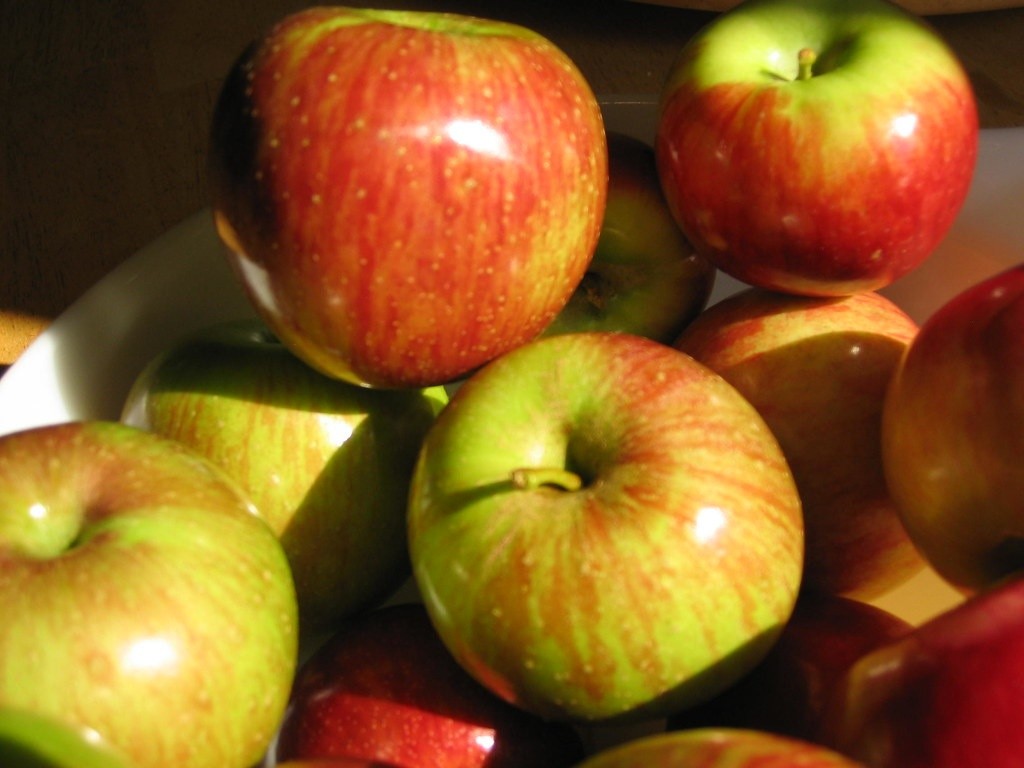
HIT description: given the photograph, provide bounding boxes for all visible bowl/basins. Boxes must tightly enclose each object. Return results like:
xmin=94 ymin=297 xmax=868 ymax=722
xmin=0 ymin=133 xmax=1024 ymax=768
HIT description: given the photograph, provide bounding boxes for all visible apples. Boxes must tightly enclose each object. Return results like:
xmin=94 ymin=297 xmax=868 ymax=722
xmin=0 ymin=0 xmax=1024 ymax=768
